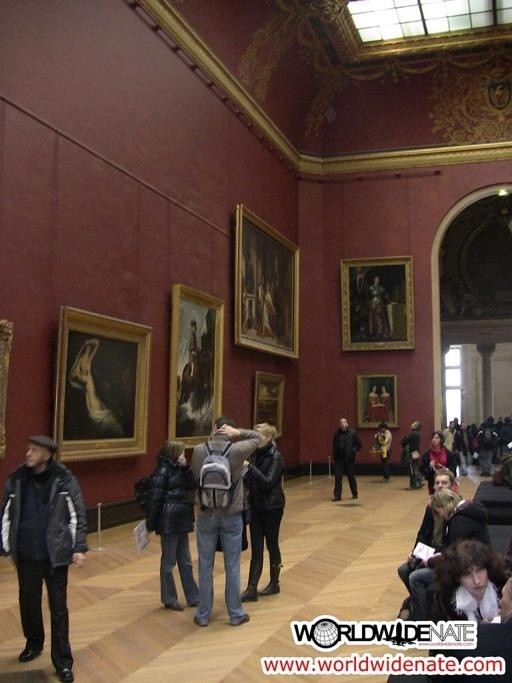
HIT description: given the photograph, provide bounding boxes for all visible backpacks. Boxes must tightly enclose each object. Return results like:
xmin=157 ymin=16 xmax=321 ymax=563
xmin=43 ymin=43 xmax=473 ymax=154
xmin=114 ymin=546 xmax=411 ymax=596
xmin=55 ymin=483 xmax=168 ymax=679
xmin=199 ymin=440 xmax=234 ymax=508
xmin=483 ymin=427 xmax=493 ymax=446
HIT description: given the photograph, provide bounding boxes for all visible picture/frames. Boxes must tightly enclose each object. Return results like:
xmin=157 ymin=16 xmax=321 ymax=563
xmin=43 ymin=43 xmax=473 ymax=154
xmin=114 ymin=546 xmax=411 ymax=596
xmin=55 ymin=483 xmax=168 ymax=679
xmin=167 ymin=283 xmax=226 ymax=446
xmin=252 ymin=369 xmax=286 ymax=439
xmin=339 ymin=255 xmax=416 ymax=354
xmin=233 ymin=203 xmax=301 ymax=360
xmin=0 ymin=320 xmax=15 ymax=459
xmin=52 ymin=305 xmax=153 ymax=463
xmin=356 ymin=373 xmax=399 ymax=430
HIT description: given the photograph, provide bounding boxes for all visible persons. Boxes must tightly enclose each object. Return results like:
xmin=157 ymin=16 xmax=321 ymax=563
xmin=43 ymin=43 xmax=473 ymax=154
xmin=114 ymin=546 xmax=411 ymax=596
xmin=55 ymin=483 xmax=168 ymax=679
xmin=0 ymin=435 xmax=89 ymax=682
xmin=145 ymin=440 xmax=204 ymax=611
xmin=330 ymin=417 xmax=362 ymax=501
xmin=235 ymin=423 xmax=285 ymax=601
xmin=387 ymin=415 xmax=512 ymax=683
xmin=358 ymin=274 xmax=393 ymax=338
xmin=67 ymin=338 xmax=126 ymax=439
xmin=381 ymin=385 xmax=393 ymax=421
xmin=188 ymin=320 xmax=200 ymax=377
xmin=374 ymin=423 xmax=392 ymax=482
xmin=367 ymin=385 xmax=380 ymax=420
xmin=191 ymin=417 xmax=263 ymax=626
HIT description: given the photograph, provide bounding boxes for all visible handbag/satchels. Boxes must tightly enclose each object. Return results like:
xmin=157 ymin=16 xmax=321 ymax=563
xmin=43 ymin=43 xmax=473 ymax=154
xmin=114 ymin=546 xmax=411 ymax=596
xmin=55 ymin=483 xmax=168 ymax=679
xmin=410 ymin=450 xmax=420 ymax=460
xmin=134 ymin=473 xmax=151 ymax=512
xmin=493 ymin=469 xmax=504 ymax=485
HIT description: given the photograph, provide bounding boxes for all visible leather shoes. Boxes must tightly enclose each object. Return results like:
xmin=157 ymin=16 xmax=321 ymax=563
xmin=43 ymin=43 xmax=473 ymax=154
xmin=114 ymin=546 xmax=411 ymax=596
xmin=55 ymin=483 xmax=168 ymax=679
xmin=56 ymin=668 xmax=74 ymax=682
xmin=20 ymin=646 xmax=43 ymax=661
xmin=164 ymin=601 xmax=200 ymax=610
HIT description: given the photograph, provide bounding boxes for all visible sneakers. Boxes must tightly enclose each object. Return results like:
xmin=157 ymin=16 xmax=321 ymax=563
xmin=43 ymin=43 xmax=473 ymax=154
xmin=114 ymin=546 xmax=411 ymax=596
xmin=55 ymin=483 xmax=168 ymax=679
xmin=407 ymin=480 xmax=425 ymax=490
xmin=331 ymin=497 xmax=341 ymax=501
xmin=479 ymin=473 xmax=492 ymax=476
xmin=194 ymin=616 xmax=207 ymax=626
xmin=231 ymin=613 xmax=249 ymax=626
xmin=353 ymin=494 xmax=357 ymax=499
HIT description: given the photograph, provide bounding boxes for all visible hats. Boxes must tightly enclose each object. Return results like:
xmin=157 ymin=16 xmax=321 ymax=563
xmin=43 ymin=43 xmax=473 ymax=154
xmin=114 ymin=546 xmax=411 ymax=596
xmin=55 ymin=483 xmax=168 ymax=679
xmin=26 ymin=435 xmax=58 ymax=452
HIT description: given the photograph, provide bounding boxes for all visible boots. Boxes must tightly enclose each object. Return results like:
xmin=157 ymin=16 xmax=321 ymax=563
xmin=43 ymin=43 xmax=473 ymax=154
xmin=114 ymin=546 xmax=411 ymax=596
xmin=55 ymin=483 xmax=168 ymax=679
xmin=258 ymin=557 xmax=283 ymax=595
xmin=240 ymin=558 xmax=263 ymax=602
